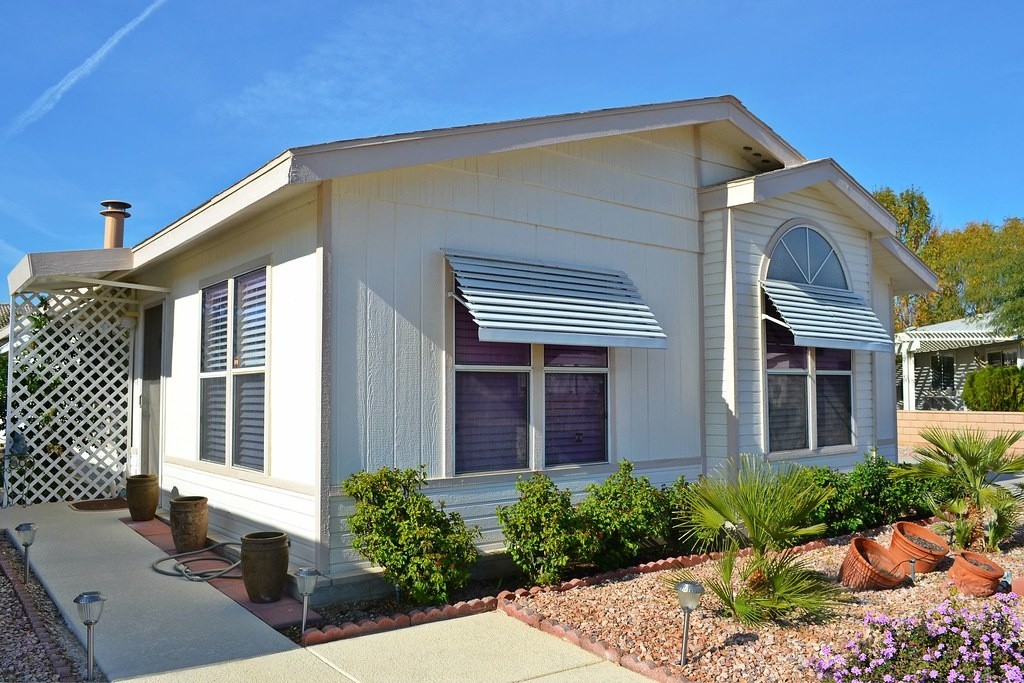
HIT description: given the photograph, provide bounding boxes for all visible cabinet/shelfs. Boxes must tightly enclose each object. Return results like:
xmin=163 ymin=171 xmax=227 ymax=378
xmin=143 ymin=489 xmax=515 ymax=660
xmin=2 ymin=454 xmax=35 ymax=508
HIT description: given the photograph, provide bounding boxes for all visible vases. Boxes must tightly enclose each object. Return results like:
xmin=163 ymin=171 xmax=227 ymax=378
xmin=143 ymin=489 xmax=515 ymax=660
xmin=838 ymin=537 xmax=905 ymax=590
xmin=890 ymin=522 xmax=950 ymax=573
xmin=947 ymin=552 xmax=1005 ymax=597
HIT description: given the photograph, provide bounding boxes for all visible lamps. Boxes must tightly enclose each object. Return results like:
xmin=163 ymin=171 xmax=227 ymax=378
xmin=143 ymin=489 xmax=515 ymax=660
xmin=672 ymin=580 xmax=704 ymax=666
xmin=15 ymin=523 xmax=41 ymax=583
xmin=293 ymin=565 xmax=320 ymax=635
xmin=73 ymin=591 xmax=108 ymax=682
